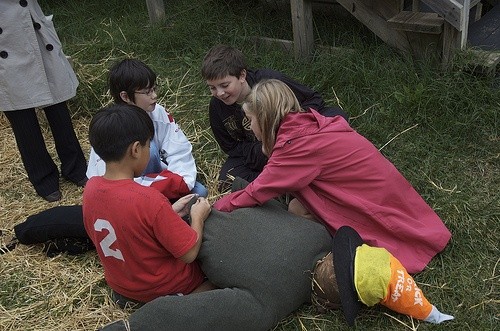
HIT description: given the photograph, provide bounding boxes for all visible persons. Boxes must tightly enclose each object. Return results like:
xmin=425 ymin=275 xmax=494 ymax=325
xmin=85 ymin=57 xmax=208 ymax=206
xmin=211 ymin=79 xmax=451 ymax=273
xmin=201 ymin=44 xmax=352 ymax=204
xmin=80 ymin=102 xmax=212 ymax=310
xmin=0 ymin=0 xmax=89 ymax=203
xmin=14 ymin=185 xmax=391 ymax=331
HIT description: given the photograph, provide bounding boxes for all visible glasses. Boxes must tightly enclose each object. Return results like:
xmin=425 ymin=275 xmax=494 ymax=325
xmin=133 ymin=84 xmax=160 ymax=96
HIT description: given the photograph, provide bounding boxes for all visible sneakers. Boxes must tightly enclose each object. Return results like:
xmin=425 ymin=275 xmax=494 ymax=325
xmin=76 ymin=177 xmax=88 ymax=188
xmin=46 ymin=189 xmax=62 ymax=201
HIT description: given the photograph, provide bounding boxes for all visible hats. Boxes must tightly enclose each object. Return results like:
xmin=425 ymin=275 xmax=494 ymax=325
xmin=332 ymin=225 xmax=455 ymax=327
